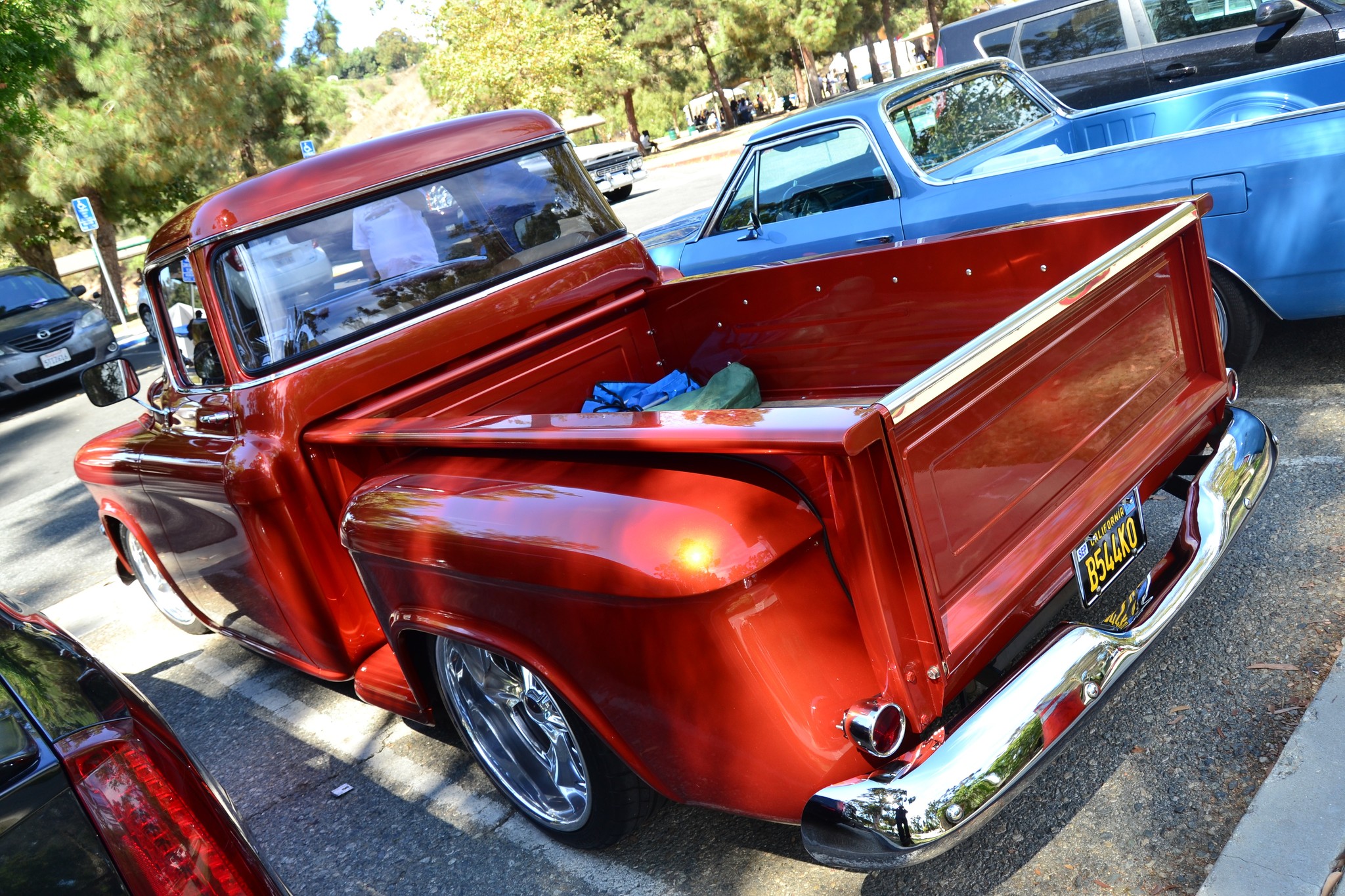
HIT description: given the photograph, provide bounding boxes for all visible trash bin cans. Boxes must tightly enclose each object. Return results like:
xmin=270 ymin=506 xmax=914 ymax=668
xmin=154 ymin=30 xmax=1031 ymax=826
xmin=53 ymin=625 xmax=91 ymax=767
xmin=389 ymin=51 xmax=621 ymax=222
xmin=688 ymin=125 xmax=696 ymax=135
xmin=668 ymin=128 xmax=678 ymax=140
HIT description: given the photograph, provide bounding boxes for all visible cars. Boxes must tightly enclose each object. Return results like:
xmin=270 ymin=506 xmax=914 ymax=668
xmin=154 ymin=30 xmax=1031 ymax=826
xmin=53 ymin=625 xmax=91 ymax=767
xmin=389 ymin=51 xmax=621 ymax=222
xmin=0 ymin=596 xmax=293 ymax=895
xmin=430 ymin=141 xmax=649 ymax=229
xmin=136 ymin=234 xmax=337 ymax=347
xmin=0 ymin=266 xmax=122 ymax=396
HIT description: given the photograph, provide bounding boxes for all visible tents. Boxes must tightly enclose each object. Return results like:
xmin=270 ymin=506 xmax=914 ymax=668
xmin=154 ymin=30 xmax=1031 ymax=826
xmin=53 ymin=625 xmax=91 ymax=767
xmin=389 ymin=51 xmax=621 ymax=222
xmin=830 ymin=19 xmax=948 ymax=84
xmin=559 ymin=109 xmax=606 ymax=143
xmin=46 ymin=232 xmax=152 ymax=278
xmin=682 ymin=71 xmax=773 ymax=113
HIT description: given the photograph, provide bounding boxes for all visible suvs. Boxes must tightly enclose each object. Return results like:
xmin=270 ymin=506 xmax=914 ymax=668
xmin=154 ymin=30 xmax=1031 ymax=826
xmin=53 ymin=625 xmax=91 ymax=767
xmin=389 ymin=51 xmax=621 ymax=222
xmin=933 ymin=0 xmax=1344 ymax=144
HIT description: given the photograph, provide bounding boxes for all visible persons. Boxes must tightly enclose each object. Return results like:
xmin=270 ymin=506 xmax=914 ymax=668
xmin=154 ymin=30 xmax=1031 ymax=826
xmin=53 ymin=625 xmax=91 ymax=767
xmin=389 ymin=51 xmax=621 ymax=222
xmin=910 ymin=48 xmax=942 ymax=70
xmin=814 ymin=64 xmax=854 ymax=100
xmin=61 ymin=263 xmax=144 ymax=311
xmin=352 ymin=194 xmax=440 ymax=284
xmin=688 ymin=92 xmax=767 ymax=133
xmin=638 ymin=128 xmax=663 ymax=155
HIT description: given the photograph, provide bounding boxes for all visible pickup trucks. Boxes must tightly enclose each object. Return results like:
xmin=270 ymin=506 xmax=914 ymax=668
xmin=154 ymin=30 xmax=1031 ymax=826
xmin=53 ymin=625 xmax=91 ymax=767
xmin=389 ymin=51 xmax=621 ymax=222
xmin=75 ymin=118 xmax=1281 ymax=874
xmin=644 ymin=57 xmax=1343 ymax=379
